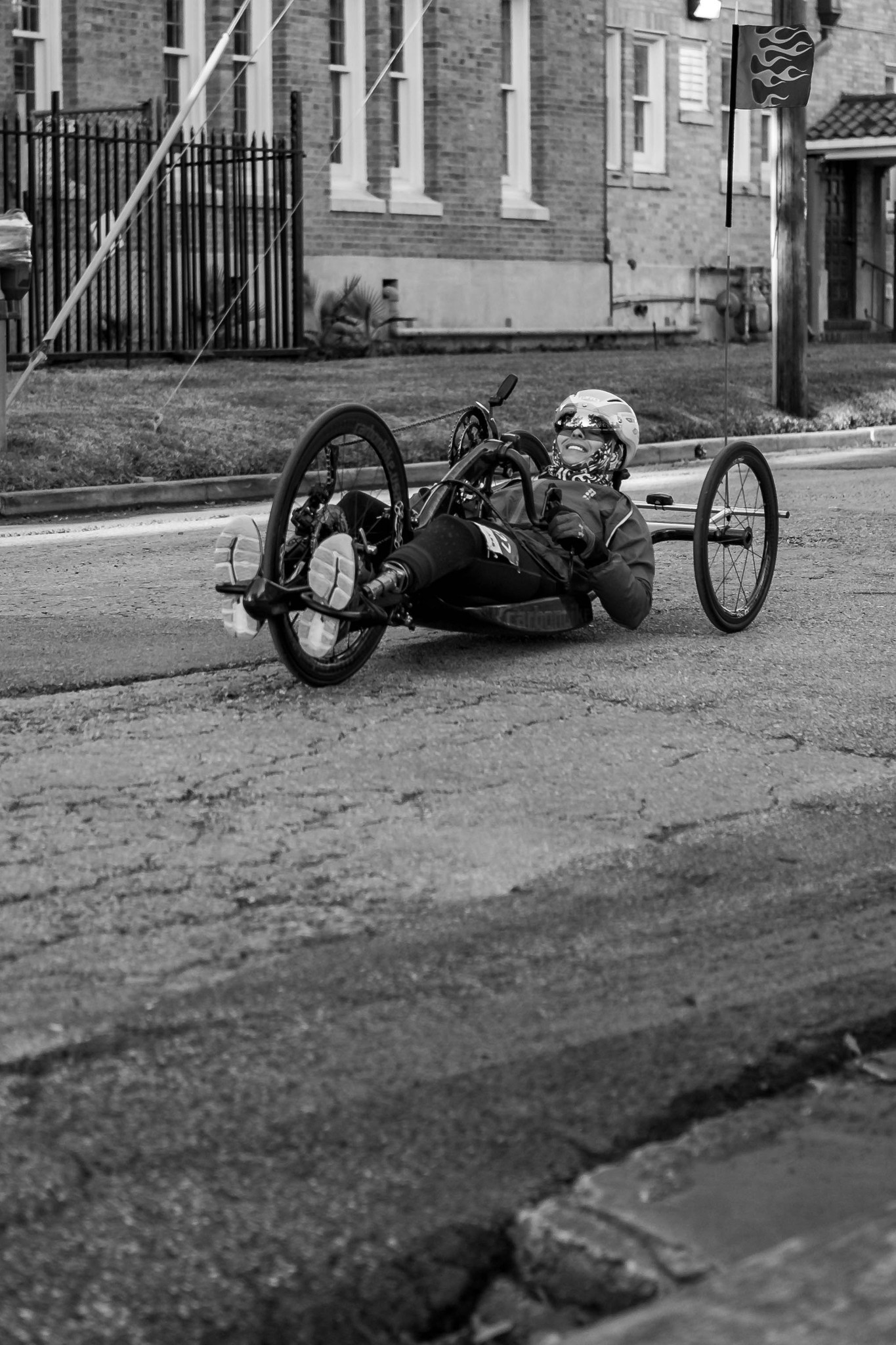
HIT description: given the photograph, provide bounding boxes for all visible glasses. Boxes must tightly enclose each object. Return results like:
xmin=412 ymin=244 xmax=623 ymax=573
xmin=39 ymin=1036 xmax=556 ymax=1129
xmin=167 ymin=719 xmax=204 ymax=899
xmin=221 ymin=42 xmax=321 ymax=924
xmin=561 ymin=419 xmax=606 ymax=437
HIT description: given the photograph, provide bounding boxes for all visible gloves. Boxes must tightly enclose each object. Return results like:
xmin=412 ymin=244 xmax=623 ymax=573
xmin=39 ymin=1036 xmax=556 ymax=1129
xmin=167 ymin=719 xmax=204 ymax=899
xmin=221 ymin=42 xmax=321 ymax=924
xmin=543 ymin=506 xmax=608 ymax=567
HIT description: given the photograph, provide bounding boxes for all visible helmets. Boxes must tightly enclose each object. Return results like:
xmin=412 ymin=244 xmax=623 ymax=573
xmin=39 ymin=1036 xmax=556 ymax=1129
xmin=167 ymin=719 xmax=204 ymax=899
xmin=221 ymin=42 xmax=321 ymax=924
xmin=553 ymin=389 xmax=640 ymax=472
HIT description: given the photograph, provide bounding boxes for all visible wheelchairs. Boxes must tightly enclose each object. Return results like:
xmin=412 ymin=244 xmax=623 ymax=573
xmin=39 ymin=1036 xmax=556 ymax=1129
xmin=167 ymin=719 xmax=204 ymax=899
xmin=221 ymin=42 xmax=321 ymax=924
xmin=215 ymin=373 xmax=790 ymax=689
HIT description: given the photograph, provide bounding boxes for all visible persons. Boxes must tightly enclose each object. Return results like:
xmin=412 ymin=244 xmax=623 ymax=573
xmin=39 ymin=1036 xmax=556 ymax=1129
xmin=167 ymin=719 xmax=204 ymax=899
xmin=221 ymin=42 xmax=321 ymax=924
xmin=215 ymin=389 xmax=656 ymax=659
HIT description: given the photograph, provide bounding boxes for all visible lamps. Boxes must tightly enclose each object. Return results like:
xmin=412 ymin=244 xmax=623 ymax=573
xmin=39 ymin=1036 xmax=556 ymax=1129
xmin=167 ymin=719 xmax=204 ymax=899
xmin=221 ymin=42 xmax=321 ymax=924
xmin=686 ymin=0 xmax=722 ymax=21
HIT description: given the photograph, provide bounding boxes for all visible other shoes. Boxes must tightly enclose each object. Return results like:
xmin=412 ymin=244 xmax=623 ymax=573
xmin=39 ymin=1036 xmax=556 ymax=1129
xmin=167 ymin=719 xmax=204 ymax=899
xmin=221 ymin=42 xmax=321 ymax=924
xmin=213 ymin=516 xmax=268 ymax=640
xmin=297 ymin=532 xmax=358 ymax=657
